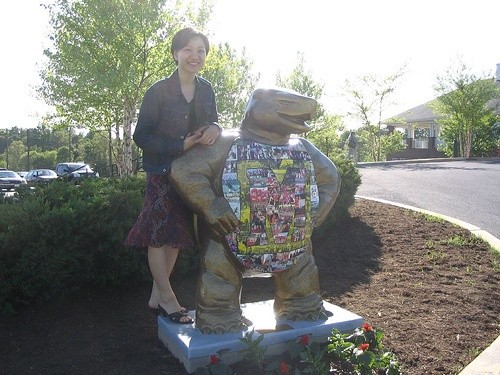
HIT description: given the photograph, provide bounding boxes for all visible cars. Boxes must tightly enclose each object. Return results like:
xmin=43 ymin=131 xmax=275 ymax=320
xmin=25 ymin=168 xmax=63 ymax=186
xmin=0 ymin=171 xmax=27 ymax=192
xmin=16 ymin=172 xmax=30 ymax=178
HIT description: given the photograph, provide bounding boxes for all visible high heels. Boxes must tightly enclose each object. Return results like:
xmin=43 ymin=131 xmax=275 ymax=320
xmin=147 ymin=304 xmax=194 ymax=324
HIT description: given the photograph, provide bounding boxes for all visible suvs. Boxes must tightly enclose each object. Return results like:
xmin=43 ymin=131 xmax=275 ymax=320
xmin=53 ymin=163 xmax=101 ymax=184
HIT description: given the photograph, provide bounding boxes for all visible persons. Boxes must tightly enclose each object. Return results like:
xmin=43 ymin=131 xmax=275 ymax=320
xmin=130 ymin=26 xmax=223 ymax=327
